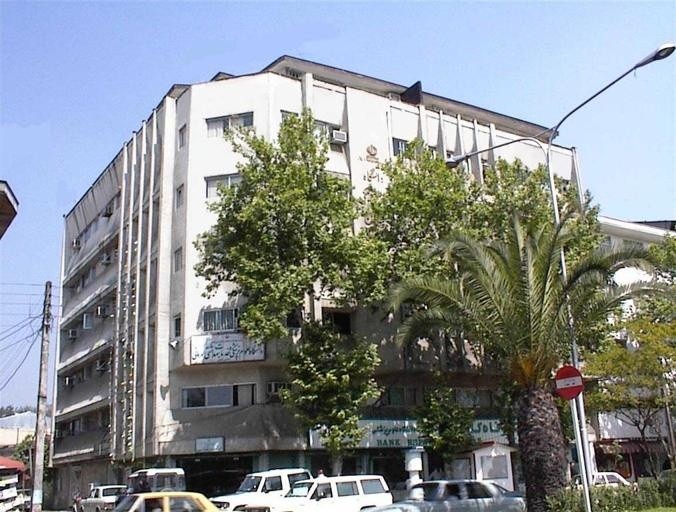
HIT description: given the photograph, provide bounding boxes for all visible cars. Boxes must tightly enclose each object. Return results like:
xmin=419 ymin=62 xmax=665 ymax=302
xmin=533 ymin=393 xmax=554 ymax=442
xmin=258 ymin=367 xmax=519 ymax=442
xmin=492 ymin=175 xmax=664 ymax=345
xmin=563 ymin=471 xmax=639 ymax=498
xmin=361 ymin=479 xmax=526 ymax=512
xmin=77 ymin=485 xmax=127 ymax=512
xmin=108 ymin=491 xmax=222 ymax=512
xmin=658 ymin=468 xmax=676 ymax=480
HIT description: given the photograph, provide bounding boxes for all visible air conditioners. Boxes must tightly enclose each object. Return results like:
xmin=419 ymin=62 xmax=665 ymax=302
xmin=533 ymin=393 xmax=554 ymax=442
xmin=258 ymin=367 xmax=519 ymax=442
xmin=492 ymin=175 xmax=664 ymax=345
xmin=329 ymin=129 xmax=348 ymax=145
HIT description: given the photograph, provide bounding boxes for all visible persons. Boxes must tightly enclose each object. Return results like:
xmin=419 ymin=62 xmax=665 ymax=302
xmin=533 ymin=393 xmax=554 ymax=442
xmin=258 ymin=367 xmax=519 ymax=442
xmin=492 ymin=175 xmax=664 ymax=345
xmin=114 ymin=479 xmax=151 ymax=506
xmin=428 ymin=466 xmax=442 ymax=480
xmin=316 ymin=468 xmax=325 ymax=477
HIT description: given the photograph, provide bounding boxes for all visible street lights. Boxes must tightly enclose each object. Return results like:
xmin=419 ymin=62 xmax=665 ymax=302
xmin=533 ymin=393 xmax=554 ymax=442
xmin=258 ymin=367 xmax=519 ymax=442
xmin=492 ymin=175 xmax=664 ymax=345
xmin=445 ymin=42 xmax=675 ymax=507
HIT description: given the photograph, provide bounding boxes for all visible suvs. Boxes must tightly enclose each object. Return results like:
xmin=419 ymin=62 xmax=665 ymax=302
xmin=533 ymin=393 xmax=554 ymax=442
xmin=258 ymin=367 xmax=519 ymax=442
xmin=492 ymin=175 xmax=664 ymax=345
xmin=207 ymin=467 xmax=314 ymax=512
xmin=115 ymin=468 xmax=186 ymax=509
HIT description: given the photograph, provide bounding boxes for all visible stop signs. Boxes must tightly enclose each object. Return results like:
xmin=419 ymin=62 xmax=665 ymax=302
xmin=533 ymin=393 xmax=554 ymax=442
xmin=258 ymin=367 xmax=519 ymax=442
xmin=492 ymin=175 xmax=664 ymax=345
xmin=555 ymin=366 xmax=584 ymax=401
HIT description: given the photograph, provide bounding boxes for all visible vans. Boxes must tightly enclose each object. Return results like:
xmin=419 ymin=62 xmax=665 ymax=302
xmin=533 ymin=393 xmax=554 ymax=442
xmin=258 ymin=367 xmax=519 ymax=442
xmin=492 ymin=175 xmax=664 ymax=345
xmin=244 ymin=474 xmax=393 ymax=511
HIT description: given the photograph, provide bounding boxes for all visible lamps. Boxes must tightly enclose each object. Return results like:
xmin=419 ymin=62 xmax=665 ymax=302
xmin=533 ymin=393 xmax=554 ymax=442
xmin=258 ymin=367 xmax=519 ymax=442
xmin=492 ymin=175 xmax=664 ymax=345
xmin=167 ymin=338 xmax=181 ymax=351
